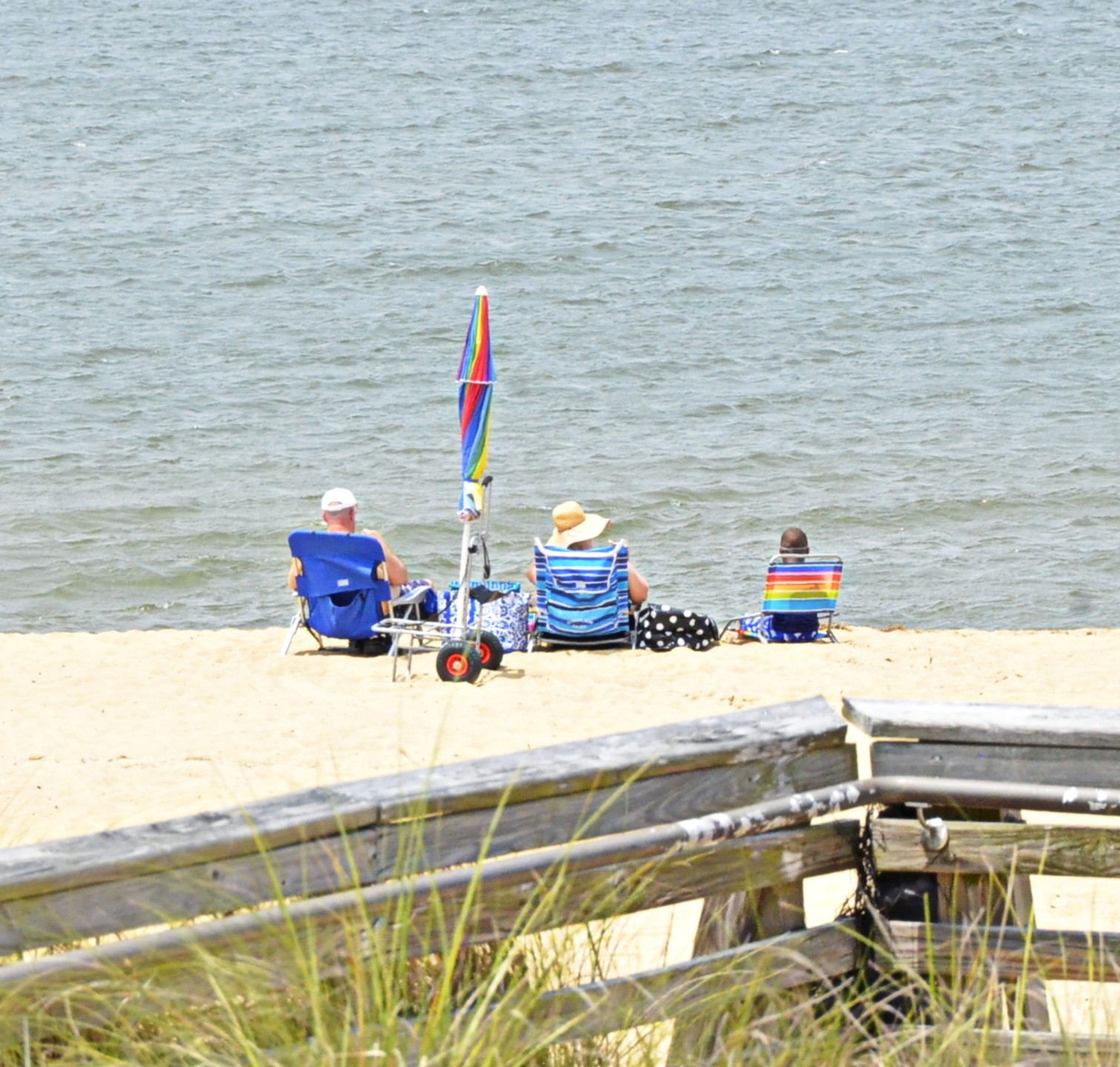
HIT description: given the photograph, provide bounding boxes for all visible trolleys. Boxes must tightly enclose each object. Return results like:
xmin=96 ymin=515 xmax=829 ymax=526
xmin=373 ymin=477 xmax=504 ymax=684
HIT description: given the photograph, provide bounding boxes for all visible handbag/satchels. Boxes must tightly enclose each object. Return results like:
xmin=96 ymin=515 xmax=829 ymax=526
xmin=637 ymin=603 xmax=720 ymax=652
xmin=437 ymin=581 xmax=532 ymax=654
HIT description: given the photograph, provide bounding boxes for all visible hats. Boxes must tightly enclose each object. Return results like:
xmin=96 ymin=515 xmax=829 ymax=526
xmin=543 ymin=500 xmax=612 ymax=549
xmin=321 ymin=488 xmax=356 ymax=512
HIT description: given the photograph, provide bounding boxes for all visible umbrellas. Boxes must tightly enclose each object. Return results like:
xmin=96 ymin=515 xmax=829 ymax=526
xmin=450 ymin=285 xmax=497 ymax=644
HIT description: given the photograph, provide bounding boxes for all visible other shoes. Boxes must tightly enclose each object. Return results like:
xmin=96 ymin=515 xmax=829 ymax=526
xmin=349 ymin=637 xmax=403 ymax=657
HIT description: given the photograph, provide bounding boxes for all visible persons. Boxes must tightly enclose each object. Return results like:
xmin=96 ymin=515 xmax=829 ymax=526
xmin=735 ymin=527 xmax=819 ymax=641
xmin=287 ymin=488 xmax=436 ymax=626
xmin=526 ymin=500 xmax=649 ymax=641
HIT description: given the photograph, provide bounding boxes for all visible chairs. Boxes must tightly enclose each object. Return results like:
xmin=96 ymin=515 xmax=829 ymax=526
xmin=278 ymin=530 xmax=432 ymax=652
xmin=719 ymin=553 xmax=844 ymax=642
xmin=532 ymin=535 xmax=638 ymax=651
